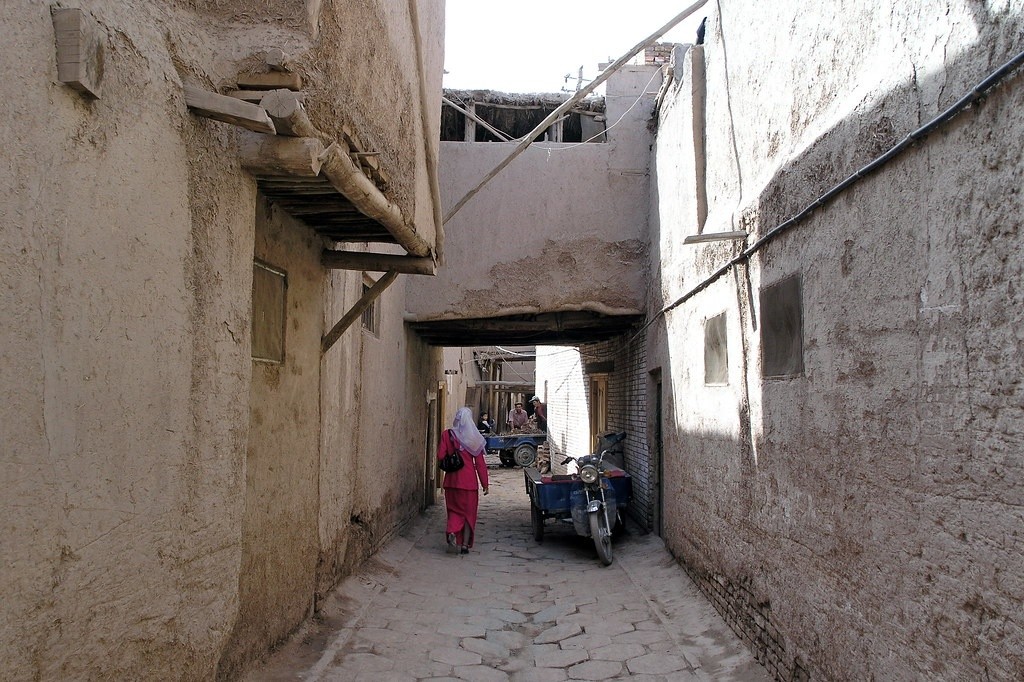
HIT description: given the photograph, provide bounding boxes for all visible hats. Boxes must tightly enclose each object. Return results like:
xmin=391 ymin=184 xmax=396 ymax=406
xmin=514 ymin=402 xmax=523 ymax=405
xmin=529 ymin=396 xmax=539 ymax=402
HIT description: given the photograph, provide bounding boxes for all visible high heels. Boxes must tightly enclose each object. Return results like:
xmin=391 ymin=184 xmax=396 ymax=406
xmin=461 ymin=545 xmax=469 ymax=554
xmin=447 ymin=534 xmax=455 ymax=547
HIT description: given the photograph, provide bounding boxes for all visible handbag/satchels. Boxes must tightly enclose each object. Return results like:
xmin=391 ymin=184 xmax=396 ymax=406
xmin=438 ymin=429 xmax=464 ymax=471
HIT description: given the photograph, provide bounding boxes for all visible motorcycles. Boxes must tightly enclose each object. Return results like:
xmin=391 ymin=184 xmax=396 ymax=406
xmin=523 ymin=450 xmax=632 ymax=566
xmin=480 ymin=430 xmax=548 ymax=467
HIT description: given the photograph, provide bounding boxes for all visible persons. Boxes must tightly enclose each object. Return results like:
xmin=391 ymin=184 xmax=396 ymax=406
xmin=438 ymin=407 xmax=488 ymax=555
xmin=509 ymin=402 xmax=528 ymax=434
xmin=477 ymin=411 xmax=491 ymax=433
xmin=529 ymin=396 xmax=547 ymax=433
